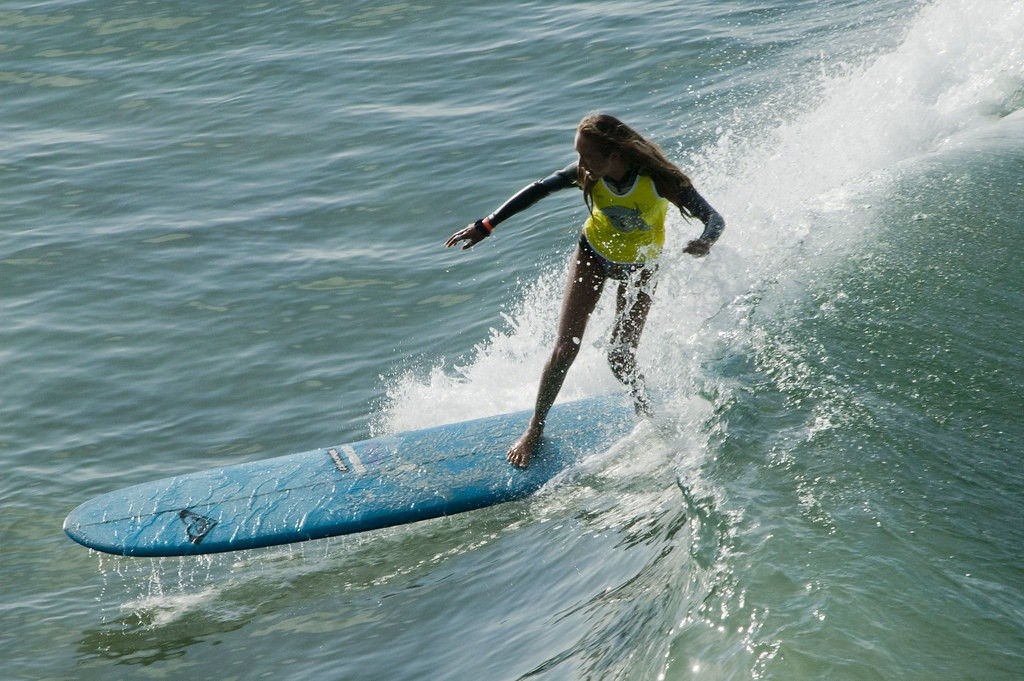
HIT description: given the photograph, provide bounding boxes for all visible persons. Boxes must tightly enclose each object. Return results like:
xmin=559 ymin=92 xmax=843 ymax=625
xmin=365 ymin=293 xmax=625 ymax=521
xmin=444 ymin=114 xmax=726 ymax=471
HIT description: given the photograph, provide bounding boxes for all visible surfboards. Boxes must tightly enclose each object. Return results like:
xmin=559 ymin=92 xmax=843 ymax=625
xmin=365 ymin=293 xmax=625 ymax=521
xmin=64 ymin=388 xmax=659 ymax=552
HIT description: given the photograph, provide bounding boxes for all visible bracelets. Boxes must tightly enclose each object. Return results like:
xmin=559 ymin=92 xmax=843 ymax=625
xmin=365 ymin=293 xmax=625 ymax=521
xmin=475 ymin=219 xmax=491 ymax=237
xmin=482 ymin=219 xmax=493 ymax=231
